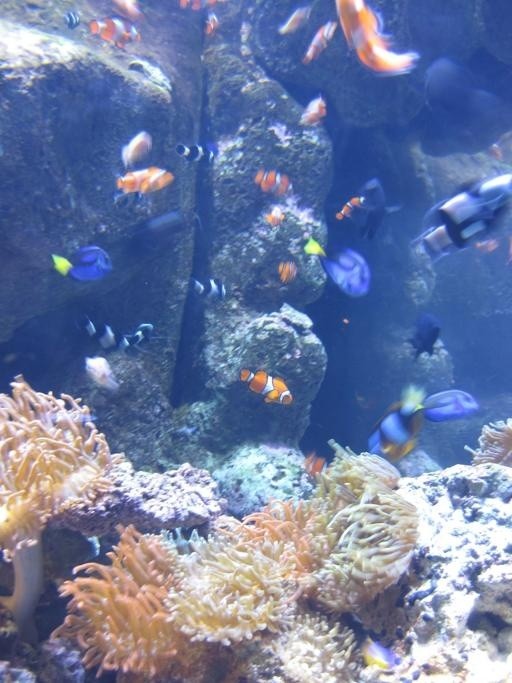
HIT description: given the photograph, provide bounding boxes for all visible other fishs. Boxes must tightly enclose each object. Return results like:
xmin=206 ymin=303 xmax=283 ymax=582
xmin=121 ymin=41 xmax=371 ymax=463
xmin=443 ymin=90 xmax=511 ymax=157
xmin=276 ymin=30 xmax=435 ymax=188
xmin=50 ymin=246 xmax=111 ymax=283
xmin=194 ymin=280 xmax=232 ymax=301
xmin=84 ymin=358 xmax=118 ymax=393
xmin=237 ymin=371 xmax=292 ymax=408
xmin=277 ymin=263 xmax=300 ymax=287
xmin=304 ymin=240 xmax=369 ymax=299
xmin=80 ymin=317 xmax=153 ymax=353
xmin=48 ymin=0 xmax=420 ymax=249
